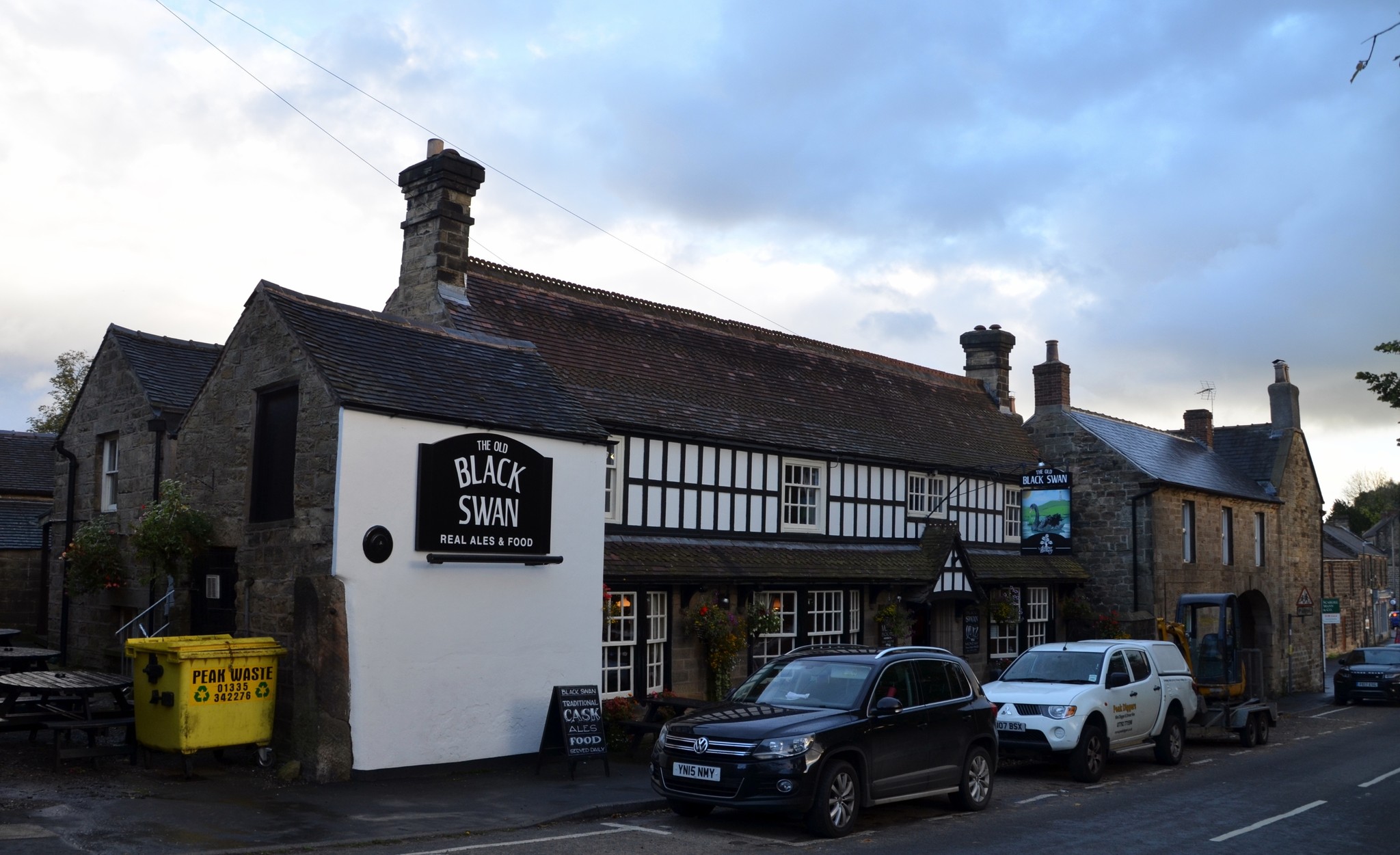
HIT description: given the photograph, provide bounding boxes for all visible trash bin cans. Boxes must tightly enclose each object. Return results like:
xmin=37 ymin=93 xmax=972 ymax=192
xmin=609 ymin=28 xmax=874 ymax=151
xmin=124 ymin=631 xmax=288 ymax=753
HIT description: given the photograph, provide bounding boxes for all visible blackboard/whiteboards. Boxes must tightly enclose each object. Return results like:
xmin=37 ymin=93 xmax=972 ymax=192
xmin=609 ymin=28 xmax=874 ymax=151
xmin=539 ymin=685 xmax=609 ymax=763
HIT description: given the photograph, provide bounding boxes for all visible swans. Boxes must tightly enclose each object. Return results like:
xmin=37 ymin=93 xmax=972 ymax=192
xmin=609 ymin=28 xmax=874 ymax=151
xmin=1028 ymin=501 xmax=1066 ymax=533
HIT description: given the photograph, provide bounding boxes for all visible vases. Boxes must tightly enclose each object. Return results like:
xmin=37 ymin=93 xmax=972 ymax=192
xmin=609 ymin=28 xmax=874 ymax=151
xmin=754 ymin=618 xmax=770 ymax=629
xmin=1065 ymin=613 xmax=1080 ymax=619
xmin=1082 ymin=609 xmax=1096 ymax=621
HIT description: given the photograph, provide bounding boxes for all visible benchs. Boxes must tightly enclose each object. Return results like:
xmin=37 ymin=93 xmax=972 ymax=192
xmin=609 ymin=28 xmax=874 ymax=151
xmin=0 ymin=693 xmax=137 ymax=765
xmin=618 ymin=718 xmax=661 ymax=732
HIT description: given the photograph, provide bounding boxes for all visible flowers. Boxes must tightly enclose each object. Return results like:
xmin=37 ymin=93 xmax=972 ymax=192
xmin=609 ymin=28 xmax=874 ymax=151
xmin=54 ymin=514 xmax=128 ymax=603
xmin=605 ymin=696 xmax=640 ymax=749
xmin=123 ymin=474 xmax=215 ymax=592
xmin=682 ymin=594 xmax=747 ymax=701
xmin=1063 ymin=593 xmax=1096 ymax=612
xmin=602 ymin=583 xmax=620 ymax=626
xmin=869 ymin=599 xmax=917 ymax=646
xmin=990 ymin=585 xmax=1025 ymax=628
xmin=748 ymin=599 xmax=784 ymax=639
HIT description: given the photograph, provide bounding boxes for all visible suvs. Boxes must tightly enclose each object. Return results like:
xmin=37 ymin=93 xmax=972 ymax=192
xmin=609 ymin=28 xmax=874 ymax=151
xmin=648 ymin=643 xmax=1000 ymax=839
xmin=981 ymin=639 xmax=1200 ymax=784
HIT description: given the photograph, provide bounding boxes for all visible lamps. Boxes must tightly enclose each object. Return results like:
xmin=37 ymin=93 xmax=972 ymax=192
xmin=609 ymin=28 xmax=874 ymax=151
xmin=616 ymin=594 xmax=631 ymax=608
xmin=773 ymin=598 xmax=783 ymax=609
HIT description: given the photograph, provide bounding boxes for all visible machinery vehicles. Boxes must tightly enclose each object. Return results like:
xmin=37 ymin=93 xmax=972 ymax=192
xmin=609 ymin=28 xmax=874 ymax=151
xmin=1154 ymin=580 xmax=1279 ymax=749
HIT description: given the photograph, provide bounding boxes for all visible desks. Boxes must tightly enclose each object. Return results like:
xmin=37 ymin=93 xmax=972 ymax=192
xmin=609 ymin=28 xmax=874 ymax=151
xmin=625 ymin=693 xmax=713 ymax=758
xmin=0 ymin=669 xmax=133 ymax=763
xmin=0 ymin=644 xmax=62 ymax=673
xmin=0 ymin=628 xmax=21 ymax=646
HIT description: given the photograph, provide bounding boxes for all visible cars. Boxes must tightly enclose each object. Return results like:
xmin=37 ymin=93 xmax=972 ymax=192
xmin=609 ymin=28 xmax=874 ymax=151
xmin=1332 ymin=643 xmax=1400 ymax=708
xmin=1388 ymin=610 xmax=1400 ymax=630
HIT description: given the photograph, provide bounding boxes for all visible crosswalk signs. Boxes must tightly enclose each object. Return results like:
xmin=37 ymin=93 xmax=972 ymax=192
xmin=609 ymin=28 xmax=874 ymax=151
xmin=1295 ymin=585 xmax=1314 ymax=607
